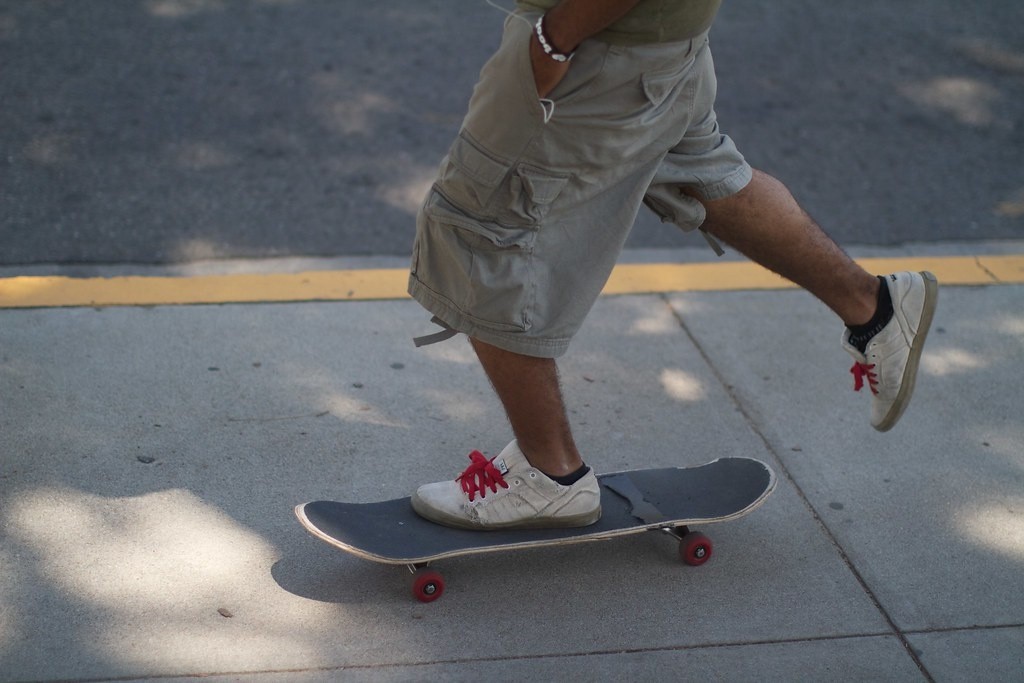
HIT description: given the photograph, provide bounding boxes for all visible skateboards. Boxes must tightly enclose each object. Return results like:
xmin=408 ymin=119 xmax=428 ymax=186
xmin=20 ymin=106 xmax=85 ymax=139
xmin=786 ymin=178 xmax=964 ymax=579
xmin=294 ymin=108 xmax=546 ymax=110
xmin=292 ymin=456 xmax=781 ymax=605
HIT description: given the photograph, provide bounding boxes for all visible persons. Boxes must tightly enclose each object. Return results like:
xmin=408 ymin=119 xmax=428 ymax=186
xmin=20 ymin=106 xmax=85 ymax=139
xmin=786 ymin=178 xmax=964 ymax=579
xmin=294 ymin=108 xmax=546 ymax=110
xmin=407 ymin=0 xmax=938 ymax=530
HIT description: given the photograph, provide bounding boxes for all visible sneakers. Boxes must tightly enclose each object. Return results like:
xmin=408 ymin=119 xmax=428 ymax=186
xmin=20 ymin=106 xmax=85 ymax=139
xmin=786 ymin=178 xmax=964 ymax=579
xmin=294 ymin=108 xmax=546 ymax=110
xmin=412 ymin=438 xmax=603 ymax=530
xmin=842 ymin=270 xmax=939 ymax=431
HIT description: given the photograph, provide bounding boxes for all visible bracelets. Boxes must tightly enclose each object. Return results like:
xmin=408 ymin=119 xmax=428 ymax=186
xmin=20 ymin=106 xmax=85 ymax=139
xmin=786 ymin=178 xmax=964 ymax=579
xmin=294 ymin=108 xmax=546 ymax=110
xmin=534 ymin=12 xmax=575 ymax=63
